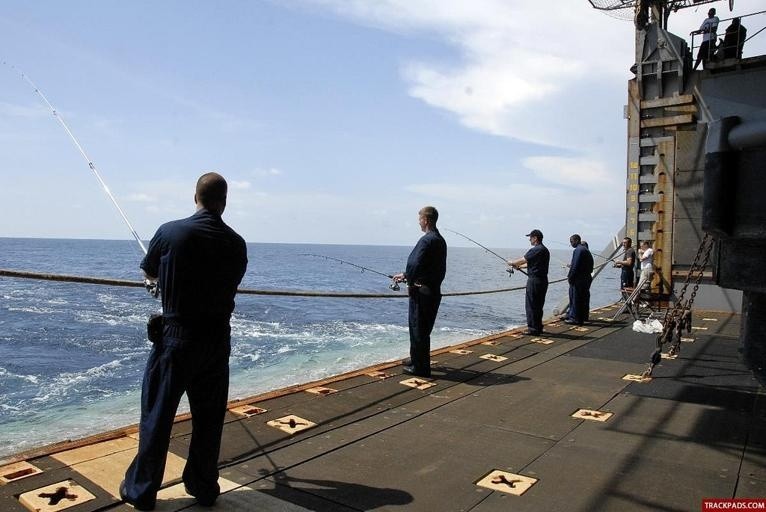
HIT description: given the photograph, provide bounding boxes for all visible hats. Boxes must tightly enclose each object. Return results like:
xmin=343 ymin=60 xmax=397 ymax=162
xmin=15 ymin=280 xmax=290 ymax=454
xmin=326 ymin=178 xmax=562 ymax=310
xmin=526 ymin=229 xmax=543 ymax=238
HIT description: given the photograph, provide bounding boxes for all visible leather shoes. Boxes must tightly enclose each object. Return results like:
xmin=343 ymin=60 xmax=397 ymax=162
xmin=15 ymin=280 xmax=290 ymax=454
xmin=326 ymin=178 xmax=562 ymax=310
xmin=402 ymin=365 xmax=431 ymax=378
xmin=119 ymin=479 xmax=156 ymax=511
xmin=185 ymin=486 xmax=217 ymax=506
xmin=559 ymin=316 xmax=569 ymax=321
xmin=522 ymin=330 xmax=540 ymax=336
xmin=564 ymin=319 xmax=580 ymax=324
xmin=402 ymin=358 xmax=412 ymax=366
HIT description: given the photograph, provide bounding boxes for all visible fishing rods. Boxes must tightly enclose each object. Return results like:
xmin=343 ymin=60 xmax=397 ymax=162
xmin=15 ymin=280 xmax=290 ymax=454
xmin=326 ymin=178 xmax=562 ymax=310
xmin=0 ymin=59 xmax=162 ymax=295
xmin=227 ymin=407 xmax=288 ymax=480
xmin=591 ymin=251 xmax=614 ymax=263
xmin=301 ymin=254 xmax=409 ymax=292
xmin=443 ymin=228 xmax=530 ymax=276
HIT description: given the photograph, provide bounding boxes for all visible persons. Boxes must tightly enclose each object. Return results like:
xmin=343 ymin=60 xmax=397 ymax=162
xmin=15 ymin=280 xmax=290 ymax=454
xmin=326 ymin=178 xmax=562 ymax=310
xmin=564 ymin=233 xmax=591 ymax=330
xmin=722 ymin=17 xmax=747 ymax=59
xmin=581 ymin=240 xmax=594 ymax=323
xmin=506 ymin=229 xmax=550 ymax=340
xmin=691 ymin=8 xmax=719 ymax=71
xmin=638 ymin=240 xmax=655 ymax=291
xmin=392 ymin=205 xmax=447 ymax=379
xmin=119 ymin=171 xmax=248 ymax=511
xmin=614 ymin=237 xmax=636 ymax=304
